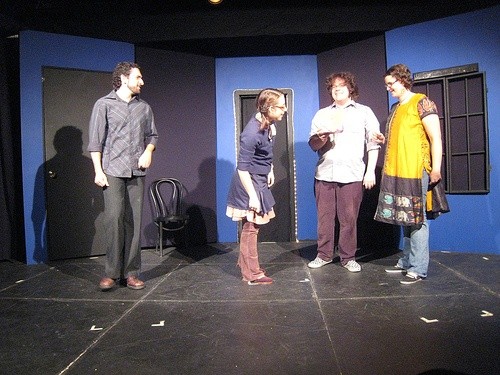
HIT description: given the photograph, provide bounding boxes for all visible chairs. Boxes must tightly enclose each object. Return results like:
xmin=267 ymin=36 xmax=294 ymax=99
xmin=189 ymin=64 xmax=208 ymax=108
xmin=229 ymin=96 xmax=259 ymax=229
xmin=150 ymin=177 xmax=191 ymax=256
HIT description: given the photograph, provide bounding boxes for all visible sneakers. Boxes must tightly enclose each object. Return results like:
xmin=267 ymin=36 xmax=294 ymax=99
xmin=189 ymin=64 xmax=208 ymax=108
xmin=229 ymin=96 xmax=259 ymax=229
xmin=400 ymin=272 xmax=424 ymax=284
xmin=384 ymin=264 xmax=407 ymax=272
xmin=343 ymin=260 xmax=362 ymax=272
xmin=307 ymin=256 xmax=332 ymax=268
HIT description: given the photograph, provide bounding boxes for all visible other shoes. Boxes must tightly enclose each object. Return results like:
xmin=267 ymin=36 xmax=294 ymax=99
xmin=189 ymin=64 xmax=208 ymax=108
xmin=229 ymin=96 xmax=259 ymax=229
xmin=242 ymin=269 xmax=265 ymax=281
xmin=248 ymin=275 xmax=273 ymax=285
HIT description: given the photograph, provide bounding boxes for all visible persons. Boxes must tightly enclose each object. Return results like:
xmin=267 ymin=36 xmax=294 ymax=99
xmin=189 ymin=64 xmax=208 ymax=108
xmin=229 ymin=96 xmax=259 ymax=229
xmin=307 ymin=73 xmax=380 ymax=270
xmin=226 ymin=88 xmax=288 ymax=284
xmin=88 ymin=62 xmax=158 ymax=289
xmin=373 ymin=65 xmax=442 ymax=283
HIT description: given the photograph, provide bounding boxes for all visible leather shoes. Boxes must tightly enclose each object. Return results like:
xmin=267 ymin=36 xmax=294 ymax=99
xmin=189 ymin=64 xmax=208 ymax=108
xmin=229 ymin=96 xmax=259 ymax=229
xmin=119 ymin=275 xmax=146 ymax=289
xmin=98 ymin=277 xmax=121 ymax=289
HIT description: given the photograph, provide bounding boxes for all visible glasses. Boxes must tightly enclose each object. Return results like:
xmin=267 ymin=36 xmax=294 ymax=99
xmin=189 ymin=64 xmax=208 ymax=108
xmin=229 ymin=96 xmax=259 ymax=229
xmin=384 ymin=79 xmax=397 ymax=88
xmin=329 ymin=83 xmax=347 ymax=89
xmin=267 ymin=105 xmax=287 ymax=111
